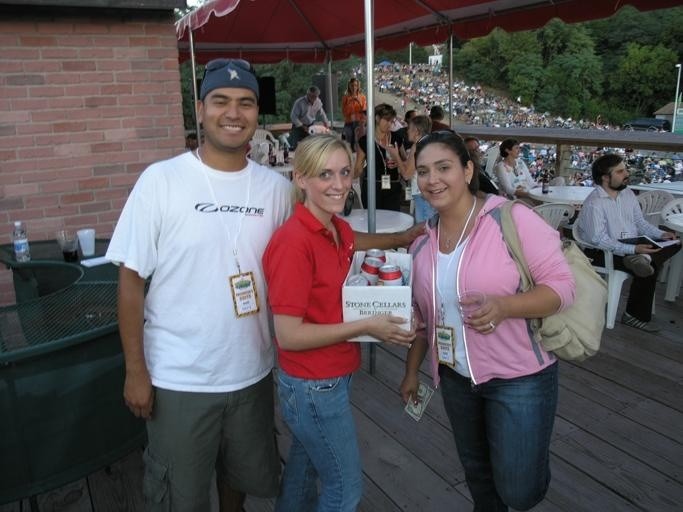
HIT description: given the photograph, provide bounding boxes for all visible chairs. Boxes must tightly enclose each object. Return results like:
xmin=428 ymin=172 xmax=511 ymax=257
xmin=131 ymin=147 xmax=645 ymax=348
xmin=250 ymin=129 xmax=279 ymax=155
xmin=635 ymin=190 xmax=674 ymax=231
xmin=573 ymin=215 xmax=656 ymax=329
xmin=532 ymin=201 xmax=576 ymax=246
xmin=659 ymin=197 xmax=683 ymax=283
xmin=309 ymin=125 xmax=329 ymax=135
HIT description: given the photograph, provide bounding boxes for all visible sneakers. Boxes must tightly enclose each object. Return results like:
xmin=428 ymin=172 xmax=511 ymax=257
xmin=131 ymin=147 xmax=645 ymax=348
xmin=623 ymin=254 xmax=655 ymax=277
xmin=621 ymin=311 xmax=663 ymax=333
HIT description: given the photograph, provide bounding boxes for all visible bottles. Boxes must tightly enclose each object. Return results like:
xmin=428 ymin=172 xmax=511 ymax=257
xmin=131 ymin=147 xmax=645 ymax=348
xmin=542 ymin=169 xmax=549 ymax=193
xmin=359 ymin=257 xmax=384 ymax=287
xmin=284 ymin=143 xmax=288 ymax=163
xmin=347 ymin=274 xmax=370 ymax=287
xmin=268 ymin=144 xmax=273 ymax=163
xmin=378 ymin=264 xmax=404 ymax=287
xmin=13 ymin=221 xmax=31 ymax=262
xmin=365 ymin=248 xmax=386 ymax=263
xmin=272 ymin=155 xmax=276 ymax=166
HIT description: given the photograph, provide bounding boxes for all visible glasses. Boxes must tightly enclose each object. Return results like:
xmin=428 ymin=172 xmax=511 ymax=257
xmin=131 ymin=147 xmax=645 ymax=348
xmin=203 ymin=58 xmax=252 ymax=71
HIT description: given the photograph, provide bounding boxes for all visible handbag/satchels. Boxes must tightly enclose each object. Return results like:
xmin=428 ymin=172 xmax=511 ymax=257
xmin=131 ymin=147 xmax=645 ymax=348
xmin=500 ymin=198 xmax=609 ymax=363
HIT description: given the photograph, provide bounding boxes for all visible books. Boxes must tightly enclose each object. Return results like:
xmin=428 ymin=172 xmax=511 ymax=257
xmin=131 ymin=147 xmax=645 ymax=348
xmin=618 ymin=237 xmax=679 ymax=247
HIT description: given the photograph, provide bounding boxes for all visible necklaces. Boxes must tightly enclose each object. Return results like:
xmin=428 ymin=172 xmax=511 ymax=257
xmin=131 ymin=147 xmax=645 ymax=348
xmin=439 ymin=202 xmax=474 ymax=249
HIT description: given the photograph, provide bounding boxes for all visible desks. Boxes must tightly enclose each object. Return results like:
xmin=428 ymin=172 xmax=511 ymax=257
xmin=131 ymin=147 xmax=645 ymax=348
xmin=342 ymin=208 xmax=414 ymax=234
xmin=0 ymin=236 xmax=154 ymax=509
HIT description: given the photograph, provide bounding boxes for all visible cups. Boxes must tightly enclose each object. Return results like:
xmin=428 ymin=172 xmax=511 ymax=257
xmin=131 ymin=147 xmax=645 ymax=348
xmin=455 ymin=290 xmax=486 ymax=321
xmin=55 ymin=229 xmax=79 ymax=252
xmin=77 ymin=229 xmax=96 ymax=256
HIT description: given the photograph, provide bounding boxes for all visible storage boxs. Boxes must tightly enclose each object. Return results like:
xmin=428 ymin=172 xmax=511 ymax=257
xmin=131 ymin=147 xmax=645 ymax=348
xmin=342 ymin=249 xmax=414 ymax=343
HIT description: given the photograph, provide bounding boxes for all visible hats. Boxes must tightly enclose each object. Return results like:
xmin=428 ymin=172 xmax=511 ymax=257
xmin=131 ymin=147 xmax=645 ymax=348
xmin=199 ymin=70 xmax=260 ymax=100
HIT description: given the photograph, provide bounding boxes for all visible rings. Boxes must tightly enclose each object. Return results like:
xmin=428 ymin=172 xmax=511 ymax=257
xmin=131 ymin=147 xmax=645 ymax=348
xmin=489 ymin=322 xmax=495 ymax=329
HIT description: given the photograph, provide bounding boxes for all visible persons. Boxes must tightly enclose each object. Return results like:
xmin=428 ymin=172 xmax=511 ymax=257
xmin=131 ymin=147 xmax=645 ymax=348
xmin=290 ymin=85 xmax=330 ymax=146
xmin=495 ymin=139 xmax=536 ymax=201
xmin=103 ymin=58 xmax=298 ymax=512
xmin=404 ymin=109 xmax=417 ymax=125
xmin=260 ymin=132 xmax=426 ymax=512
xmin=385 ymin=114 xmax=438 ymax=224
xmin=353 ymin=103 xmax=406 ymax=211
xmin=429 ymin=104 xmax=450 ymax=132
xmin=452 ymin=74 xmax=522 ymax=129
xmin=463 ymin=136 xmax=508 ymax=199
xmin=360 ymin=58 xmax=451 ymax=109
xmin=522 ymin=106 xmax=683 ymax=183
xmin=399 ymin=129 xmax=577 ymax=512
xmin=576 ymin=153 xmax=683 ymax=333
xmin=341 ymin=77 xmax=366 ymax=130
